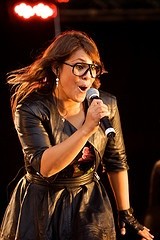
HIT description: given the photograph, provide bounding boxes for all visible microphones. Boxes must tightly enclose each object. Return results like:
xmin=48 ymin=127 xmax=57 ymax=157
xmin=86 ymin=87 xmax=117 ymax=143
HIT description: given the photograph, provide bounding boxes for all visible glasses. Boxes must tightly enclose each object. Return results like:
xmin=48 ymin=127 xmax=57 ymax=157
xmin=61 ymin=61 xmax=103 ymax=79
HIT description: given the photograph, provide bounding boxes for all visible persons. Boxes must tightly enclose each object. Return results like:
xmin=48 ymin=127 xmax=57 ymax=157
xmin=142 ymin=160 xmax=160 ymax=240
xmin=0 ymin=30 xmax=155 ymax=240
